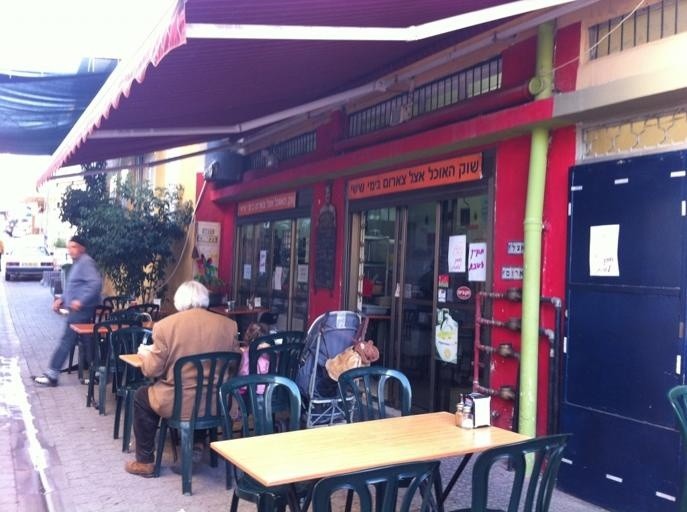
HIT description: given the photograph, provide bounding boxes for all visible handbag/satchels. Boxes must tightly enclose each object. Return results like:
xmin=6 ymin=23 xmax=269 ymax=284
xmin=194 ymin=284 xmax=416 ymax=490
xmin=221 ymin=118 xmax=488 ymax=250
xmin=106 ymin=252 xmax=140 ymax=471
xmin=326 ymin=340 xmax=382 ymax=385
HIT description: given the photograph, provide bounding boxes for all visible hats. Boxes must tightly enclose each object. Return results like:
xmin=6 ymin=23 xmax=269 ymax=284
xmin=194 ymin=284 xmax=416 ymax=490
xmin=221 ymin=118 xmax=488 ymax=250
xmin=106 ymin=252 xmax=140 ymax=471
xmin=72 ymin=236 xmax=89 ymax=248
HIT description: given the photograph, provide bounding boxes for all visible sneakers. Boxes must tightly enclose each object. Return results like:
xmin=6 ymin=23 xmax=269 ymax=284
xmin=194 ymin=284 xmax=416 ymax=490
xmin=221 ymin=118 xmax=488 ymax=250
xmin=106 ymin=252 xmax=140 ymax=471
xmin=125 ymin=461 xmax=155 ymax=478
xmin=171 ymin=442 xmax=203 ymax=475
xmin=85 ymin=377 xmax=99 ymax=385
xmin=31 ymin=376 xmax=57 ymax=386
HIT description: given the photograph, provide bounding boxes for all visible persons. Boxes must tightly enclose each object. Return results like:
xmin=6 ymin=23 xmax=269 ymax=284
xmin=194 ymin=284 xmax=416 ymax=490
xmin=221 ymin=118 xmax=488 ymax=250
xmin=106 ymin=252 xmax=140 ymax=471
xmin=31 ymin=236 xmax=103 ymax=390
xmin=234 ymin=321 xmax=279 ymax=423
xmin=124 ymin=279 xmax=245 ymax=476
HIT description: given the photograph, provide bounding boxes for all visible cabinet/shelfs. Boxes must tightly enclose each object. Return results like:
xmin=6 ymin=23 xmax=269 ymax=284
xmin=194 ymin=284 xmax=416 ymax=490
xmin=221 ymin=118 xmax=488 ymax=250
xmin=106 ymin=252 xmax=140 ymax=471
xmin=361 ymin=235 xmax=390 ymax=384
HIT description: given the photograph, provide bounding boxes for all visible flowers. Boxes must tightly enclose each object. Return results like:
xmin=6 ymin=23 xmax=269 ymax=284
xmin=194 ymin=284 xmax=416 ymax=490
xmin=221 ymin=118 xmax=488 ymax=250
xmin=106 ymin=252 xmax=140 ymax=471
xmin=194 ymin=253 xmax=224 ymax=289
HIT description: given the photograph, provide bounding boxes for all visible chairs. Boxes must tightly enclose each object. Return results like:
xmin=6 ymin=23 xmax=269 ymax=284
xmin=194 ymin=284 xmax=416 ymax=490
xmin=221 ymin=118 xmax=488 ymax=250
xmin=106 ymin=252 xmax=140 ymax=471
xmin=310 ymin=459 xmax=444 ymax=512
xmin=56 ymin=295 xmax=307 ymax=454
xmin=666 ymin=383 xmax=687 ymax=511
xmin=337 ymin=366 xmax=424 ymax=512
xmin=154 ymin=352 xmax=243 ymax=497
xmin=217 ymin=373 xmax=303 ymax=512
xmin=449 ymin=432 xmax=574 ymax=512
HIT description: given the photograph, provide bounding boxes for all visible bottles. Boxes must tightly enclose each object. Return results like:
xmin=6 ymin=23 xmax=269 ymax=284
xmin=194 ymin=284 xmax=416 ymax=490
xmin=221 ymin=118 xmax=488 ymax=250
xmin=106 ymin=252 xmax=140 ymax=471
xmin=455 ymin=402 xmax=473 ymax=429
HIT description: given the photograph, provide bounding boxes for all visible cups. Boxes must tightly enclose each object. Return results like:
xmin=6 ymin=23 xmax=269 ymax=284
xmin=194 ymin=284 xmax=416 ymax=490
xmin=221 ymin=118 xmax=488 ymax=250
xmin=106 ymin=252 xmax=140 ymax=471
xmin=228 ymin=301 xmax=236 ymax=311
